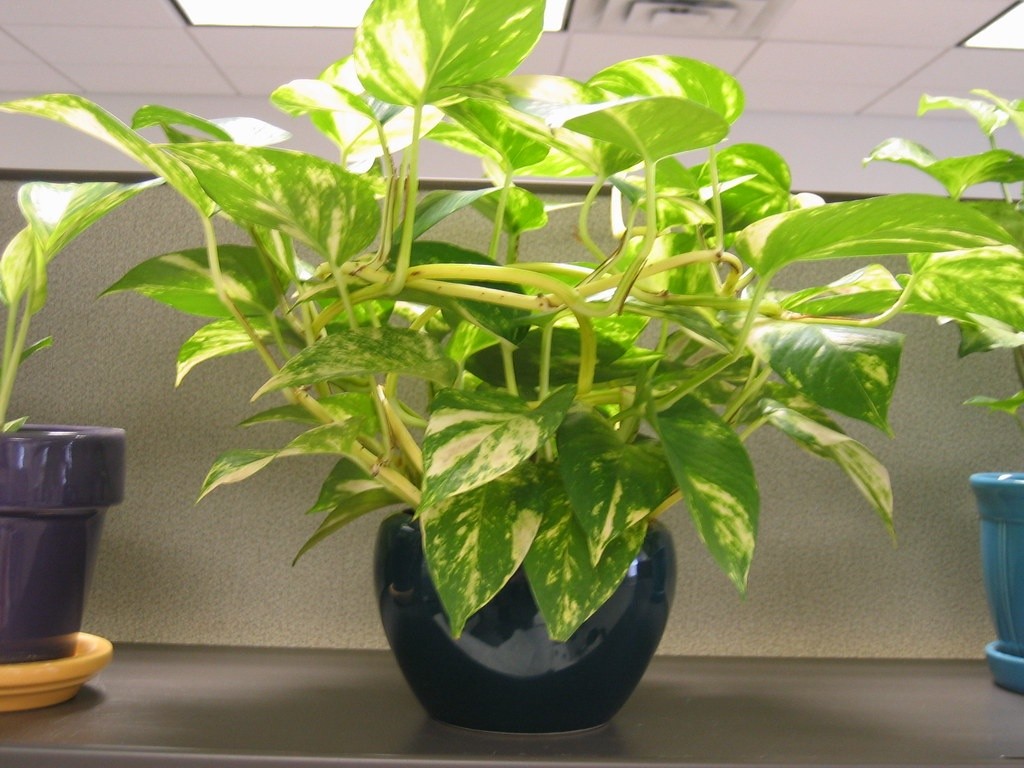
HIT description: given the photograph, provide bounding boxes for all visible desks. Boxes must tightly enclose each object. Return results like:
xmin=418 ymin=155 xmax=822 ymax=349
xmin=0 ymin=644 xmax=1024 ymax=768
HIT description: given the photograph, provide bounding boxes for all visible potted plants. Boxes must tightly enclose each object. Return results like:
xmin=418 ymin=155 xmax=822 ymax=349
xmin=0 ymin=181 xmax=128 ymax=714
xmin=0 ymin=0 xmax=1024 ymax=735
xmin=862 ymin=82 xmax=1023 ymax=692
